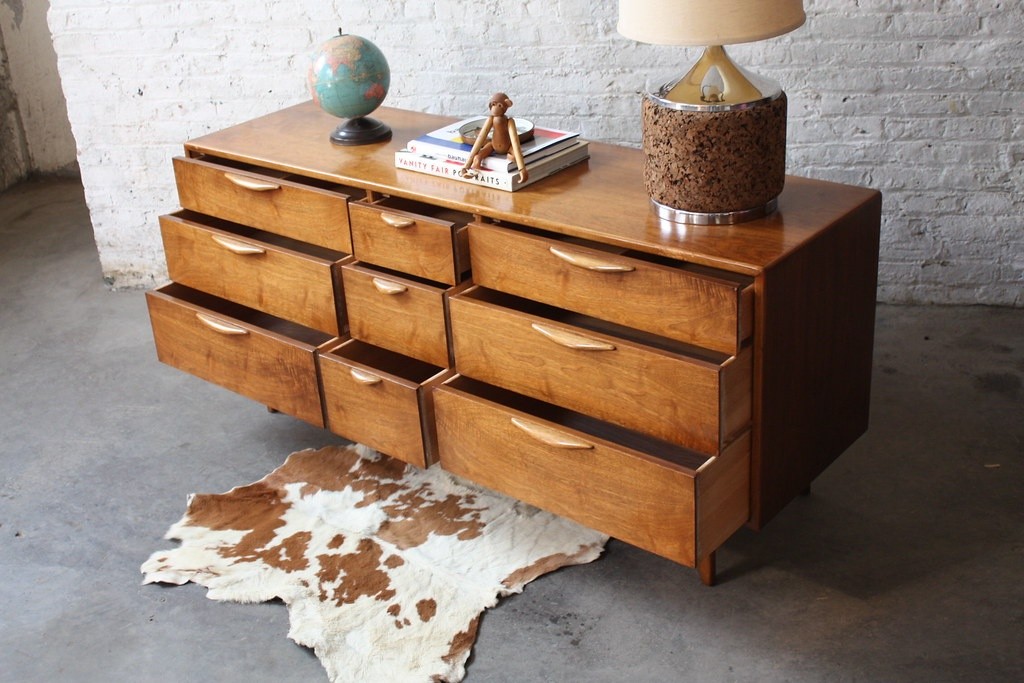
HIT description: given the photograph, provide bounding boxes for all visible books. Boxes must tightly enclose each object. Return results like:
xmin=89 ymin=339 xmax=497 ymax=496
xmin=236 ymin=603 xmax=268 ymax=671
xmin=407 ymin=115 xmax=580 ymax=175
xmin=394 ymin=140 xmax=592 ymax=193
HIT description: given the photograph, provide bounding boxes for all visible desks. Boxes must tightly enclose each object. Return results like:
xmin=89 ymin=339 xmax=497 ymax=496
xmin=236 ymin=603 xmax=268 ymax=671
xmin=145 ymin=104 xmax=881 ymax=589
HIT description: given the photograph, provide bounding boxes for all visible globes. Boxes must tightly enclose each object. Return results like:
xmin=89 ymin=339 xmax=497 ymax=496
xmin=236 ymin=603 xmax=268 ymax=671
xmin=307 ymin=27 xmax=393 ymax=146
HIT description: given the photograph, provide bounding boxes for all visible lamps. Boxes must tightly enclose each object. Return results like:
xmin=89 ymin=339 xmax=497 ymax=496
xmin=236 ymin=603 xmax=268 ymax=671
xmin=617 ymin=0 xmax=807 ymax=227
